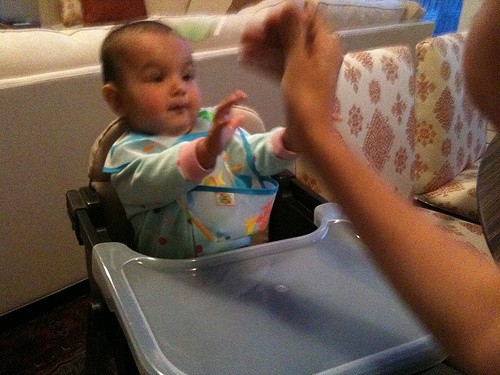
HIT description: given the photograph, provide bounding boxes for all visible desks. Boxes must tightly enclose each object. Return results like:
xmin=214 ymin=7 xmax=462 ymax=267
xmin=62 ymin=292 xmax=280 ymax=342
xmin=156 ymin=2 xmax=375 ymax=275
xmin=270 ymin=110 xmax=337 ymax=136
xmin=91 ymin=202 xmax=449 ymax=374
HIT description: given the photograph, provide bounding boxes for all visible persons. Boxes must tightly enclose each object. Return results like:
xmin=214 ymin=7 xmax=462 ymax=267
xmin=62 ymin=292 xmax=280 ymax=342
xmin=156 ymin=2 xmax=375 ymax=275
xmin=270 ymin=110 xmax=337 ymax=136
xmin=238 ymin=0 xmax=500 ymax=375
xmin=99 ymin=21 xmax=345 ymax=260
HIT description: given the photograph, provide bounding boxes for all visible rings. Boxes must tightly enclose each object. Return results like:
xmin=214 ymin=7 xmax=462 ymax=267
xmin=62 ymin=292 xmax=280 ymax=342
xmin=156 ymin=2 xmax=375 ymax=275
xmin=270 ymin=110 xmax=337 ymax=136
xmin=331 ymin=31 xmax=344 ymax=44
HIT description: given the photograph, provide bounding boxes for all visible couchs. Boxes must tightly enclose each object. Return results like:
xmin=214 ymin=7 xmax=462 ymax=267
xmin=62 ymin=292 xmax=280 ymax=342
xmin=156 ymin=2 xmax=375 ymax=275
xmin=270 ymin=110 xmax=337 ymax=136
xmin=0 ymin=0 xmax=436 ymax=318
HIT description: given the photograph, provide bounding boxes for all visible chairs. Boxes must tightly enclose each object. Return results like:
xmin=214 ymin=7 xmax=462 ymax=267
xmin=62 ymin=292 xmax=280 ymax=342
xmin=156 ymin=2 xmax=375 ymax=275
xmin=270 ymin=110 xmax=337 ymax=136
xmin=85 ymin=105 xmax=328 ymax=375
xmin=410 ymin=31 xmax=490 ymax=225
xmin=293 ymin=44 xmax=498 ymax=266
